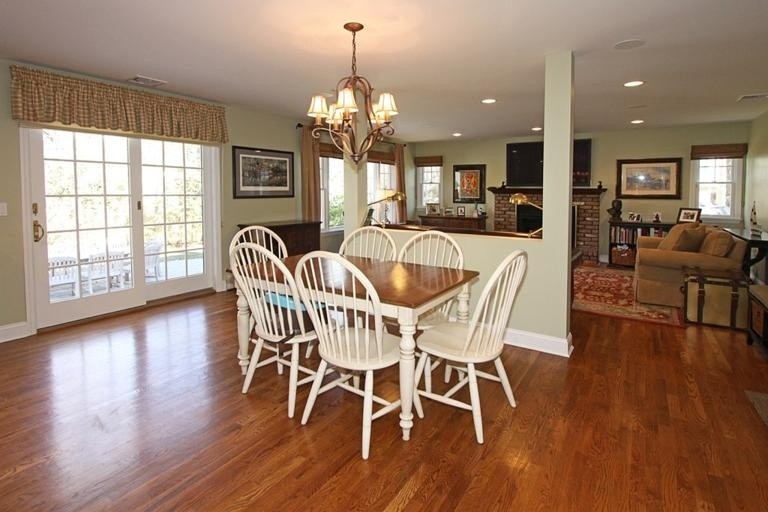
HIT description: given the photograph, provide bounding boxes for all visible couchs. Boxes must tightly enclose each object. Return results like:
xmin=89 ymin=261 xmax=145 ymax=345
xmin=634 ymin=222 xmax=747 ymax=307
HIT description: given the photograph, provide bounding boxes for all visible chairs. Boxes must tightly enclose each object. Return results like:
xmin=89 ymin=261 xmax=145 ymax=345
xmin=338 ymin=226 xmax=396 ymax=262
xmin=47 ymin=238 xmax=163 ymax=296
xmin=228 ymin=225 xmax=306 ymax=378
xmin=228 ymin=242 xmax=348 ymax=418
xmin=397 ymin=230 xmax=464 ymax=270
xmin=296 ymin=250 xmax=425 ymax=460
xmin=415 ymin=249 xmax=528 ymax=444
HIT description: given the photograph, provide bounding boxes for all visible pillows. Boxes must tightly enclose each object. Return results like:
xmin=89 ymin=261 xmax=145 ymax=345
xmin=699 ymin=229 xmax=733 ymax=256
xmin=673 ymin=225 xmax=705 ymax=252
xmin=657 ymin=221 xmax=700 ymax=249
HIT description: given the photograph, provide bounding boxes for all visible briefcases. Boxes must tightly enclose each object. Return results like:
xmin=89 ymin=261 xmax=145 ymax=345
xmin=679 ymin=274 xmax=753 ymax=331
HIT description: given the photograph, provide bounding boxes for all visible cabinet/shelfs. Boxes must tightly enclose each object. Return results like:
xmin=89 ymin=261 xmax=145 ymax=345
xmin=608 ymin=220 xmax=674 ymax=268
xmin=237 ymin=221 xmax=323 ymax=265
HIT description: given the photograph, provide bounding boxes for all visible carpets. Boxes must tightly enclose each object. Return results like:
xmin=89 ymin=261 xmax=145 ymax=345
xmin=571 ymin=257 xmax=686 ymax=328
xmin=744 ymin=389 xmax=768 ymax=428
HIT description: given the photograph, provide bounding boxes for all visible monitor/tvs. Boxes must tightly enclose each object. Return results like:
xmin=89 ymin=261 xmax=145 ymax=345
xmin=506 ymin=138 xmax=591 ymax=187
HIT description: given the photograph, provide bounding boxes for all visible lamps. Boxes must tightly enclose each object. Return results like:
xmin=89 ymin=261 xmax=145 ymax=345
xmin=307 ymin=22 xmax=399 ymax=165
xmin=358 ymin=191 xmax=406 ymax=225
xmin=509 ymin=192 xmax=543 ymax=237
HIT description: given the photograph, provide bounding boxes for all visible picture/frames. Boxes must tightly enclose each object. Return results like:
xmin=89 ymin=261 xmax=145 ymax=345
xmin=677 ymin=207 xmax=702 ymax=224
xmin=426 ymin=203 xmax=441 ymax=215
xmin=232 ymin=146 xmax=295 ymax=198
xmin=453 ymin=164 xmax=486 ymax=204
xmin=444 ymin=207 xmax=453 ymax=216
xmin=615 ymin=158 xmax=682 ymax=199
xmin=457 ymin=206 xmax=465 ymax=216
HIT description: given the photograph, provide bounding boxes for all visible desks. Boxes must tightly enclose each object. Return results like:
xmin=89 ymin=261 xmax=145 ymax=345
xmin=417 ymin=214 xmax=488 ymax=230
xmin=226 ymin=252 xmax=481 ymax=442
xmin=722 ymin=227 xmax=768 ymax=283
xmin=746 ymin=284 xmax=768 ymax=352
